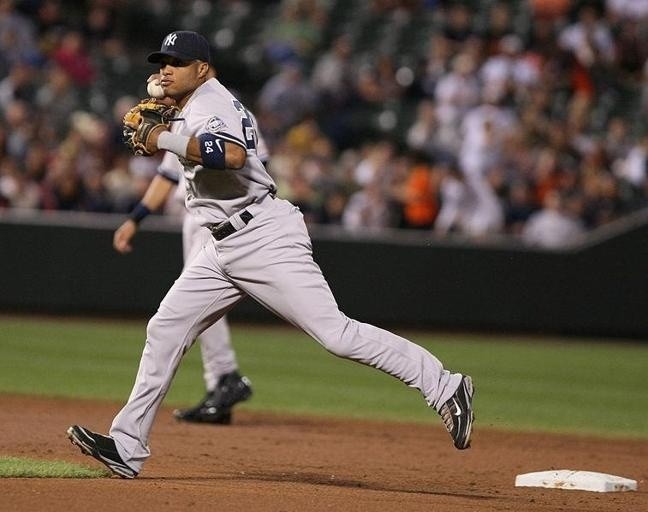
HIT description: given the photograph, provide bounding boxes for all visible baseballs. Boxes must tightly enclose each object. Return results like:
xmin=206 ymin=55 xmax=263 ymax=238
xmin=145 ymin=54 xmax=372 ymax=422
xmin=147 ymin=79 xmax=164 ymax=99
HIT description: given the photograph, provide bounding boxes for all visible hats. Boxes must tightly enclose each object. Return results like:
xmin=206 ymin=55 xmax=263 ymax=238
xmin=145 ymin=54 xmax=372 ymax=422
xmin=147 ymin=31 xmax=210 ymax=66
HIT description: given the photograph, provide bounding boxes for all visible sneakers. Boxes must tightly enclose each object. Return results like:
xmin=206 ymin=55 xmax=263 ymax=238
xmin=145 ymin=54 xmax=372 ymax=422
xmin=67 ymin=425 xmax=137 ymax=478
xmin=177 ymin=396 xmax=230 ymax=424
xmin=202 ymin=373 xmax=251 ymax=407
xmin=438 ymin=374 xmax=474 ymax=448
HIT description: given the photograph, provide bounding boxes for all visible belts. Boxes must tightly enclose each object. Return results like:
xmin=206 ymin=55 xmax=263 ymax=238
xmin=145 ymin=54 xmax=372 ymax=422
xmin=211 ymin=210 xmax=252 ymax=241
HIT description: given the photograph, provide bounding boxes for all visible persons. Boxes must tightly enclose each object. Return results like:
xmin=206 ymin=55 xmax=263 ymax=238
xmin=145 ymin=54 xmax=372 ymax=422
xmin=65 ymin=25 xmax=474 ymax=480
xmin=1 ymin=1 xmax=648 ymax=245
xmin=144 ymin=64 xmax=270 ymax=424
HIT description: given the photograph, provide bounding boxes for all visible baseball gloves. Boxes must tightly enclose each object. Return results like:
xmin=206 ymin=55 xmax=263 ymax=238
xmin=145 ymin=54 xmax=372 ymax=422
xmin=122 ymin=98 xmax=185 ymax=157
